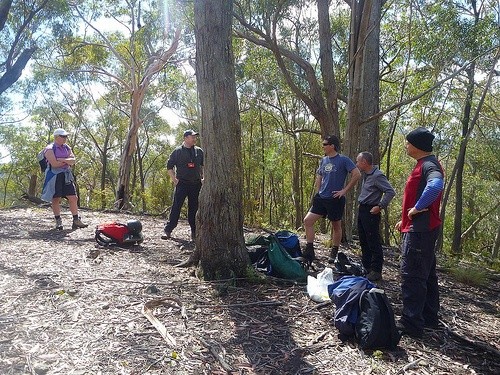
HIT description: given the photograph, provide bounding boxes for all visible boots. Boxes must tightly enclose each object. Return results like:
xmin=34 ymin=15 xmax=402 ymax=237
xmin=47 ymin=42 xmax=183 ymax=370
xmin=301 ymin=242 xmax=315 ymax=258
xmin=327 ymin=245 xmax=338 ymax=264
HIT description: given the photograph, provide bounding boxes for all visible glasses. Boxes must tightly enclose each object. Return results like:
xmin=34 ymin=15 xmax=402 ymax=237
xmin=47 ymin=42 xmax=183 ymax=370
xmin=323 ymin=143 xmax=333 ymax=146
xmin=59 ymin=135 xmax=68 ymax=138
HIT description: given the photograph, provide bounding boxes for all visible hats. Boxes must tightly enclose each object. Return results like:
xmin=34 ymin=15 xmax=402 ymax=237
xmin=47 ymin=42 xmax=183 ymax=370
xmin=183 ymin=129 xmax=200 ymax=138
xmin=405 ymin=126 xmax=436 ymax=152
xmin=54 ymin=128 xmax=70 ymax=136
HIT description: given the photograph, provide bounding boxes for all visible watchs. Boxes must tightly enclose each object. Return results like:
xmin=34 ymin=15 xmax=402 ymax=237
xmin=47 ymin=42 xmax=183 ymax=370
xmin=379 ymin=206 xmax=381 ymax=210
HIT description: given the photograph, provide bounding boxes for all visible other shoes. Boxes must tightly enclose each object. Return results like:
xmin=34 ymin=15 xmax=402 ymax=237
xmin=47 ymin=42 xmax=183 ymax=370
xmin=191 ymin=233 xmax=196 ymax=242
xmin=396 ymin=322 xmax=411 ymax=334
xmin=365 ymin=271 xmax=383 ymax=283
xmin=56 ymin=217 xmax=64 ymax=230
xmin=160 ymin=231 xmax=171 ymax=239
xmin=425 ymin=320 xmax=438 ymax=326
xmin=72 ymin=217 xmax=89 ymax=229
xmin=361 ymin=267 xmax=372 ymax=276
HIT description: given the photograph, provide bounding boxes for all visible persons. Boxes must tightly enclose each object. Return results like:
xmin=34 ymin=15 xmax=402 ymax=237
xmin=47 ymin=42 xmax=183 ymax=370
xmin=161 ymin=130 xmax=204 ymax=240
xmin=356 ymin=150 xmax=395 ymax=281
xmin=43 ymin=128 xmax=88 ymax=230
xmin=396 ymin=127 xmax=445 ymax=339
xmin=301 ymin=134 xmax=361 ymax=262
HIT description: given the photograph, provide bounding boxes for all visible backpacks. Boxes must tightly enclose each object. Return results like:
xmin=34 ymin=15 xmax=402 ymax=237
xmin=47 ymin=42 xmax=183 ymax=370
xmin=37 ymin=148 xmax=49 ymax=172
xmin=354 ymin=287 xmax=401 ymax=351
